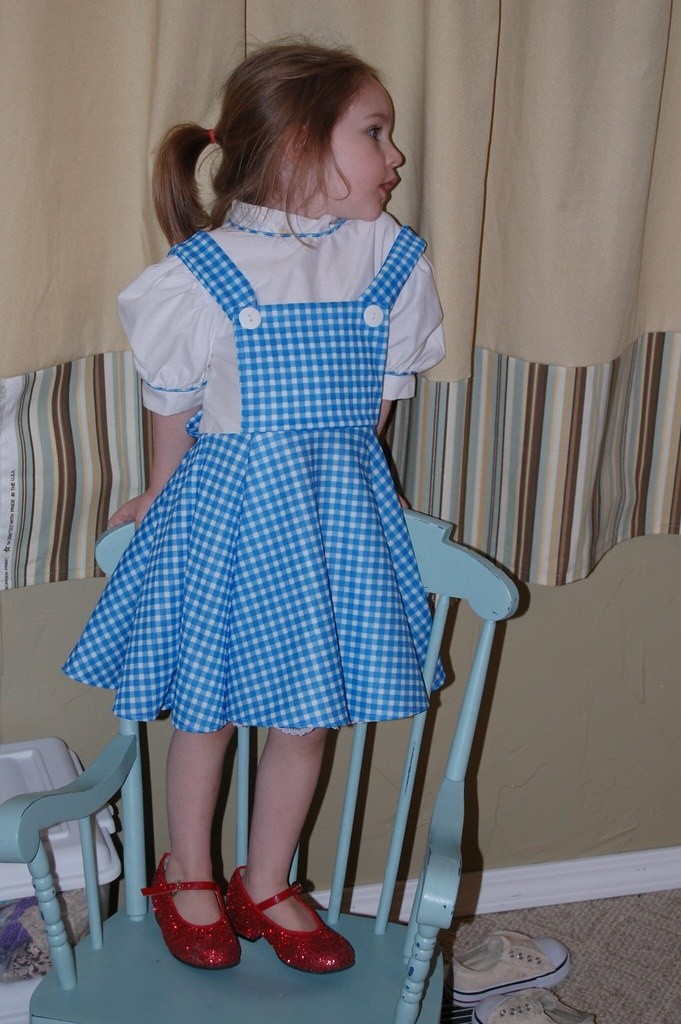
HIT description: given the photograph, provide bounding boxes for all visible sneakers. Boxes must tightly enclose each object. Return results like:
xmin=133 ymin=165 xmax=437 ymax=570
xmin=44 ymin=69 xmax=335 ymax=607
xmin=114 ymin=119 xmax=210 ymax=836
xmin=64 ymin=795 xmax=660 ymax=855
xmin=450 ymin=929 xmax=573 ymax=1006
xmin=473 ymin=987 xmax=598 ymax=1024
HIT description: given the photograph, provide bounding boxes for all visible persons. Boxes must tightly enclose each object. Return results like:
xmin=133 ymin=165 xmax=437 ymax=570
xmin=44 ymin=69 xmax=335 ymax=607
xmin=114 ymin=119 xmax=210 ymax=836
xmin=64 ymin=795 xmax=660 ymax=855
xmin=60 ymin=45 xmax=445 ymax=973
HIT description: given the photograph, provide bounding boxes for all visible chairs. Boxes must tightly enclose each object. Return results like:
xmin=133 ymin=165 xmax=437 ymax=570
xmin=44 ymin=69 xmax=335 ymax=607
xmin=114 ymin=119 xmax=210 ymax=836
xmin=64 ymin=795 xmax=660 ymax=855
xmin=1 ymin=512 xmax=521 ymax=1023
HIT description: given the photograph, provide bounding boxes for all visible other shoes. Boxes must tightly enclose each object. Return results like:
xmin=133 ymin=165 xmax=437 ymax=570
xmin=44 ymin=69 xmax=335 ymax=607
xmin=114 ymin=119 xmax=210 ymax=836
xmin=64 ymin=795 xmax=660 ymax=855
xmin=225 ymin=865 xmax=357 ymax=975
xmin=141 ymin=853 xmax=242 ymax=971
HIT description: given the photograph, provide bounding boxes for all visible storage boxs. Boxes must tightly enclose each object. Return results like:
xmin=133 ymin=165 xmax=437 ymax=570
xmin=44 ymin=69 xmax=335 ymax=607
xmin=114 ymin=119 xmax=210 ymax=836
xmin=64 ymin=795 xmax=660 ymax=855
xmin=1 ymin=738 xmax=124 ymax=954
